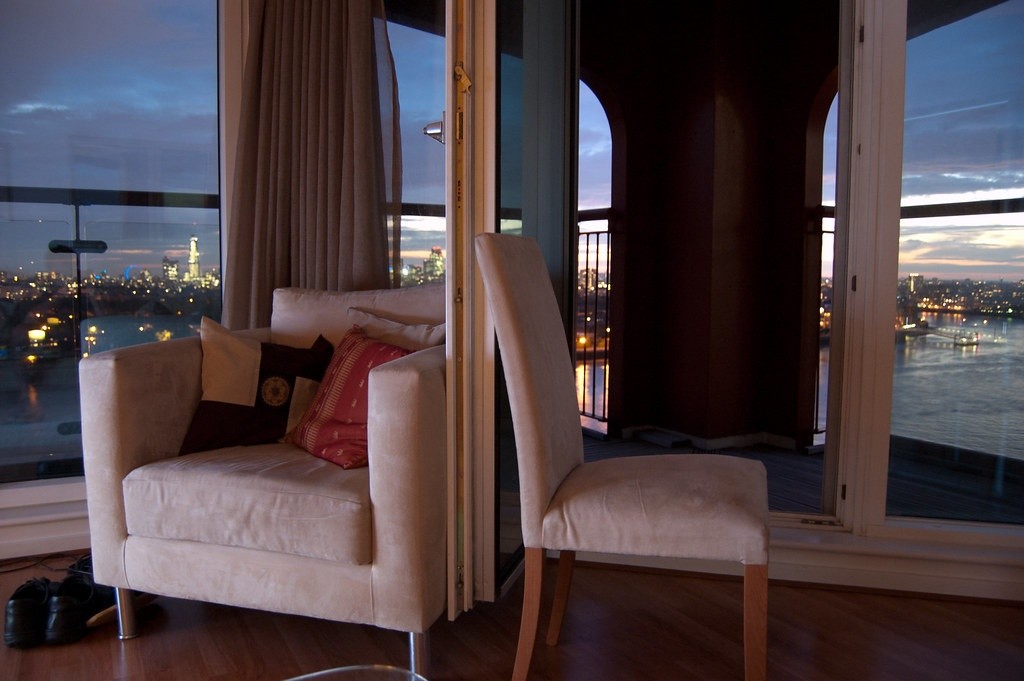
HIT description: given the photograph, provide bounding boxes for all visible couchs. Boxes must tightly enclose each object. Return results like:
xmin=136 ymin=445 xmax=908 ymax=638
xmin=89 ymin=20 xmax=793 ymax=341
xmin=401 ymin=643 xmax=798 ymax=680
xmin=77 ymin=279 xmax=448 ymax=681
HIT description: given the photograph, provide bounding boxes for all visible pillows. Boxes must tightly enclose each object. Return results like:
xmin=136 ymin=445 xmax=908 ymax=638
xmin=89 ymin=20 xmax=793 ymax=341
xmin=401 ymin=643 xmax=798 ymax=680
xmin=345 ymin=304 xmax=448 ymax=351
xmin=180 ymin=315 xmax=335 ymax=458
xmin=286 ymin=323 xmax=414 ymax=473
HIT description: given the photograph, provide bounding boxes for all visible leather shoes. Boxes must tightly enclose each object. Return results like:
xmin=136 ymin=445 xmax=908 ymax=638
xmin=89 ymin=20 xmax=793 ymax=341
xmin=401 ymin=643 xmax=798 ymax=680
xmin=44 ymin=574 xmax=115 ymax=645
xmin=6 ymin=577 xmax=66 ymax=648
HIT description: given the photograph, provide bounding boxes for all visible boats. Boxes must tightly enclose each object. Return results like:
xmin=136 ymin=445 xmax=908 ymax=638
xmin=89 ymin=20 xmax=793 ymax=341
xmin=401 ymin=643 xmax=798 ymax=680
xmin=953 ymin=333 xmax=980 ymax=346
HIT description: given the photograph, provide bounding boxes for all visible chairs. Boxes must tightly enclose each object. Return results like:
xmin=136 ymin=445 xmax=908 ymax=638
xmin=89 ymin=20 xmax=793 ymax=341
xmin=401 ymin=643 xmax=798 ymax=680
xmin=473 ymin=232 xmax=770 ymax=681
